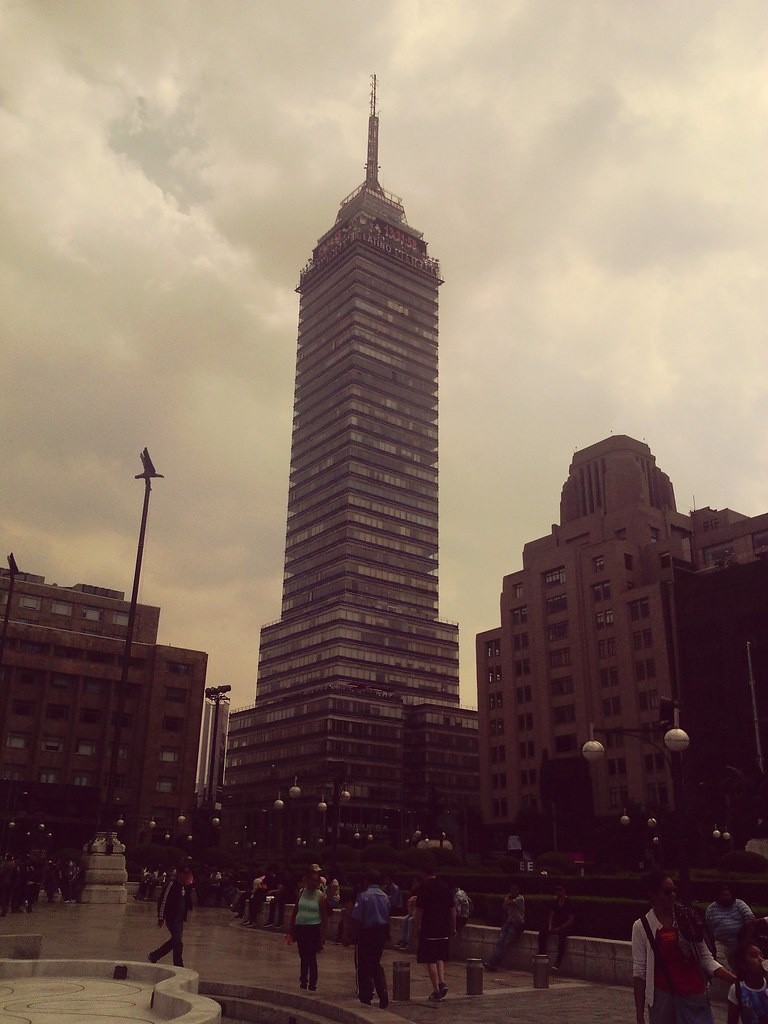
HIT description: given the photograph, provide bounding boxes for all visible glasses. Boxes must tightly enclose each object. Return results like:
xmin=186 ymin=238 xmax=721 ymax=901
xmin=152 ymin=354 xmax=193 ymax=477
xmin=555 ymin=887 xmax=565 ymax=891
xmin=181 ymin=871 xmax=190 ymax=875
xmin=657 ymin=886 xmax=680 ymax=897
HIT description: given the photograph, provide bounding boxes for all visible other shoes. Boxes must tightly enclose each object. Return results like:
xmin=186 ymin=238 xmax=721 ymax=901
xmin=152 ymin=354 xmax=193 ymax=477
xmin=379 ymin=990 xmax=388 ymax=1008
xmin=146 ymin=952 xmax=157 ymax=963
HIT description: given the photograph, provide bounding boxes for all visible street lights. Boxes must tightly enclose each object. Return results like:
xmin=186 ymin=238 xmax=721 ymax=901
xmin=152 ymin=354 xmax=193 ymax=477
xmin=204 ymin=685 xmax=231 ymax=840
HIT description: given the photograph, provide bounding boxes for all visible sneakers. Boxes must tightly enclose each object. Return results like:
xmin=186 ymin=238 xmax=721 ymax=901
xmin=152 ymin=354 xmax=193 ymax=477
xmin=428 ymin=991 xmax=442 ymax=1001
xmin=439 ymin=983 xmax=448 ymax=997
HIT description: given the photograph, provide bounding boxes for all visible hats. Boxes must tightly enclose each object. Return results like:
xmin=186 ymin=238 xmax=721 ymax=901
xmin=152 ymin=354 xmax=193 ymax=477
xmin=309 ymin=863 xmax=322 ymax=872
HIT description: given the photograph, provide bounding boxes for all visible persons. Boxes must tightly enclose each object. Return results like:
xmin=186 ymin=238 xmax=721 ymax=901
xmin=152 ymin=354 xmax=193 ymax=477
xmin=413 ymin=864 xmax=457 ymax=1002
xmin=0 ymin=832 xmax=89 ymax=917
xmin=534 ymin=883 xmax=573 ymax=972
xmin=738 ymin=916 xmax=768 ymax=948
xmin=290 ymin=872 xmax=330 ymax=991
xmin=342 ymin=869 xmax=399 ymax=1009
xmin=132 ymin=862 xmax=404 ymax=948
xmin=726 ymin=943 xmax=768 ymax=1024
xmin=632 ymin=870 xmax=737 ymax=1024
xmin=392 ymin=875 xmax=422 ymax=950
xmin=148 ymin=863 xmax=189 ymax=967
xmin=450 ymin=884 xmax=474 ymax=927
xmin=483 ymin=882 xmax=525 ymax=970
xmin=704 ymin=882 xmax=755 ymax=976
